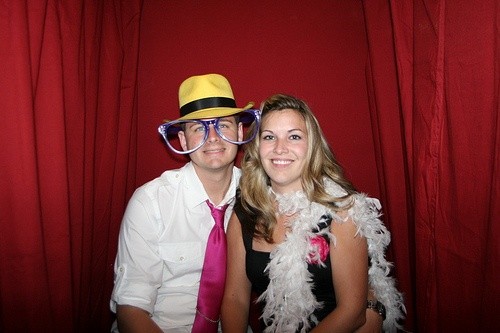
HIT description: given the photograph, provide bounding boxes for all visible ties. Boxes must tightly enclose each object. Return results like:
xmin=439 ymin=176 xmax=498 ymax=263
xmin=190 ymin=199 xmax=232 ymax=333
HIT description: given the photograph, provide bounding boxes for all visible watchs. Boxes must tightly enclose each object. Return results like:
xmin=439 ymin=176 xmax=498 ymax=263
xmin=365 ymin=299 xmax=386 ymax=322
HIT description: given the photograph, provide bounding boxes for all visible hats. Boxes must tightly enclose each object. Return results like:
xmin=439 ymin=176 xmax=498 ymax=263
xmin=158 ymin=73 xmax=254 ymax=130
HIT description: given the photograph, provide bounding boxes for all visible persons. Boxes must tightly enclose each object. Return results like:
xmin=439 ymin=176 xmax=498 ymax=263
xmin=108 ymin=76 xmax=385 ymax=333
xmin=219 ymin=93 xmax=368 ymax=333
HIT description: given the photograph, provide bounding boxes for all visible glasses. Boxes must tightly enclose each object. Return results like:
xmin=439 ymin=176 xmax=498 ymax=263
xmin=157 ymin=109 xmax=259 ymax=153
